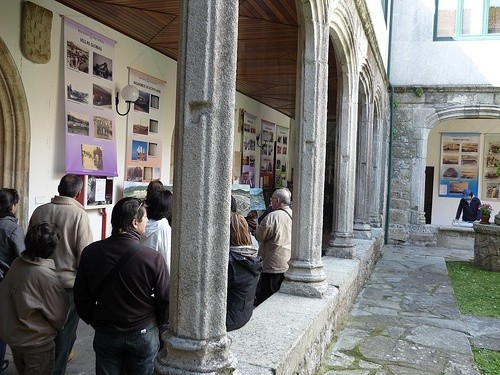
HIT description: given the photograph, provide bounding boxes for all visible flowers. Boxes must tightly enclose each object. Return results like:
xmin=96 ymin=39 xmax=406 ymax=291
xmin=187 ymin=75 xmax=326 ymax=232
xmin=477 ymin=203 xmax=494 ymax=215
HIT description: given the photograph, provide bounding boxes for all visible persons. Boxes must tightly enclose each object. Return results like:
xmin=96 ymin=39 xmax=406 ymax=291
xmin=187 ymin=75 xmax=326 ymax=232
xmin=452 ymin=189 xmax=481 ymax=224
xmin=74 ymin=197 xmax=170 ymax=375
xmin=94 ymin=154 xmax=102 ymax=169
xmin=231 ymin=186 xmax=293 ymax=232
xmin=225 ymin=212 xmax=259 ymax=332
xmin=255 ymin=187 xmax=293 ymax=297
xmin=141 ymin=178 xmax=173 ymax=271
xmin=0 ymin=220 xmax=71 ymax=375
xmin=25 ymin=173 xmax=94 ymax=375
xmin=97 ymin=62 xmax=108 ymax=78
xmin=0 ymin=188 xmax=24 ymax=267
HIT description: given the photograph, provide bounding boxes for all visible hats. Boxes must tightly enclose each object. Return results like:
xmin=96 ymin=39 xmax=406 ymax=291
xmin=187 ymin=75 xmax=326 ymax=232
xmin=461 ymin=189 xmax=471 ymax=199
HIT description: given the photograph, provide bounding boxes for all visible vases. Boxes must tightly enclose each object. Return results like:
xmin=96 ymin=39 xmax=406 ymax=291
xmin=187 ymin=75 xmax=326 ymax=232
xmin=479 ymin=214 xmax=490 ymax=224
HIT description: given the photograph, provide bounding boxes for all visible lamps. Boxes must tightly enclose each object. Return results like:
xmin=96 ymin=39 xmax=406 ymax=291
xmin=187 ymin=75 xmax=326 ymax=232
xmin=256 ymin=132 xmax=271 ymax=148
xmin=116 ymin=85 xmax=139 ymax=116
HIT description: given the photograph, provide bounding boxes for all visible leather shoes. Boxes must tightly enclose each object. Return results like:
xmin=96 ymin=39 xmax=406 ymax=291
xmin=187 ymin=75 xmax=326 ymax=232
xmin=0 ymin=360 xmax=9 ymax=372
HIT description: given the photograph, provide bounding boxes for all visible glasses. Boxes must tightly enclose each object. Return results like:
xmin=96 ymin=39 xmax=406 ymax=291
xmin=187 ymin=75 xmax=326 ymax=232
xmin=134 ymin=199 xmax=145 ymax=218
xmin=270 ymin=197 xmax=273 ymax=201
